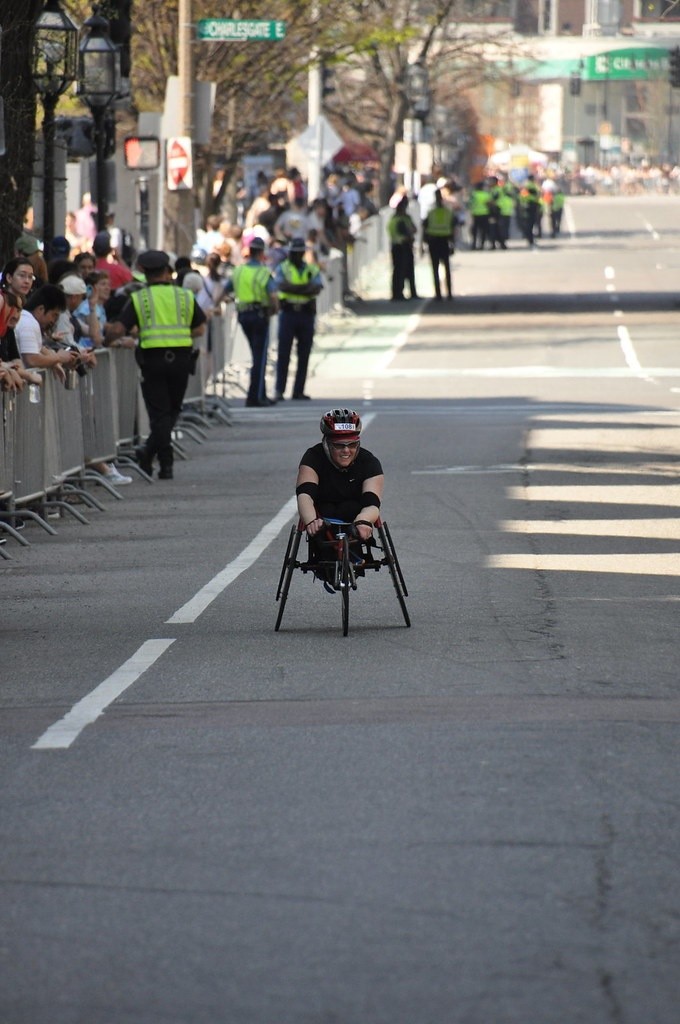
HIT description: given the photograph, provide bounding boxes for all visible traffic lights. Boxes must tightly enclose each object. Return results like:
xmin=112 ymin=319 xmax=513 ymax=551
xmin=124 ymin=136 xmax=160 ymax=170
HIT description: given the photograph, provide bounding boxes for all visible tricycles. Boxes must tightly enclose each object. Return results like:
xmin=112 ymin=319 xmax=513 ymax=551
xmin=274 ymin=516 xmax=411 ymax=635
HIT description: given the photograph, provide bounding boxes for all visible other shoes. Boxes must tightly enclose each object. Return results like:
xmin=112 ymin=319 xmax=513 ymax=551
xmin=158 ymin=469 xmax=173 ymax=479
xmin=136 ymin=446 xmax=153 ymax=477
xmin=436 ymin=293 xmax=453 ymax=301
xmin=292 ymin=392 xmax=311 ymax=400
xmin=274 ymin=393 xmax=285 ymax=401
xmin=66 ymin=495 xmax=83 ymax=505
xmin=0 ymin=537 xmax=7 ymax=545
xmin=15 ymin=520 xmax=25 ymax=530
xmin=245 ymin=394 xmax=277 ymax=408
xmin=48 ymin=511 xmax=59 ymax=518
xmin=107 ymin=463 xmax=120 ymax=475
xmin=392 ymin=293 xmax=422 ymax=303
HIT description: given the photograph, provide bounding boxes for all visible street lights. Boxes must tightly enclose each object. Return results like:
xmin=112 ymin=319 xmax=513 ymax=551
xmin=29 ymin=0 xmax=78 ymax=247
xmin=78 ymin=5 xmax=122 ymax=233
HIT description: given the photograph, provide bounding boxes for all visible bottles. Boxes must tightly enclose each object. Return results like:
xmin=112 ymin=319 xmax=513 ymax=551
xmin=64 ymin=366 xmax=76 ymax=390
xmin=29 ymin=371 xmax=41 ymax=403
xmin=77 ymin=363 xmax=93 ymax=395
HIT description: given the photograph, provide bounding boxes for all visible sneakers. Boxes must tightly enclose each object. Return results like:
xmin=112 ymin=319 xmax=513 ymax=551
xmin=102 ymin=473 xmax=132 ymax=486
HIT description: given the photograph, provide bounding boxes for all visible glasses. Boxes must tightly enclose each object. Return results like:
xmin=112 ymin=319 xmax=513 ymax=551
xmin=15 ymin=272 xmax=36 ymax=281
xmin=330 ymin=441 xmax=360 ymax=449
xmin=82 ymin=294 xmax=87 ymax=301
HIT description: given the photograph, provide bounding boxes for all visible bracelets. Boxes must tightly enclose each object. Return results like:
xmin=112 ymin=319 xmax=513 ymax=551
xmin=304 ymin=518 xmax=317 ymax=528
xmin=354 ymin=520 xmax=373 ymax=527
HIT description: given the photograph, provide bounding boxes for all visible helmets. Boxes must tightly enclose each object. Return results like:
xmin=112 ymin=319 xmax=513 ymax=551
xmin=320 ymin=408 xmax=362 ymax=435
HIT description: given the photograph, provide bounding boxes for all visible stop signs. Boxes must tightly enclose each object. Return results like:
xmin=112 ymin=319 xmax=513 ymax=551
xmin=164 ymin=137 xmax=193 ymax=190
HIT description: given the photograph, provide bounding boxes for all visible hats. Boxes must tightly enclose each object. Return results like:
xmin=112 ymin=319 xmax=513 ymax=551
xmin=92 ymin=230 xmax=111 ymax=256
xmin=138 ymin=251 xmax=169 ymax=270
xmin=45 ymin=237 xmax=70 ymax=254
xmin=250 ymin=237 xmax=265 ymax=249
xmin=14 ymin=235 xmax=44 ymax=254
xmin=58 ymin=275 xmax=87 ymax=295
xmin=288 ymin=237 xmax=306 ymax=252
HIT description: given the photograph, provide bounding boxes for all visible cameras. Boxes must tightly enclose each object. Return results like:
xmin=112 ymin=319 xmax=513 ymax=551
xmin=66 ymin=345 xmax=87 ymax=377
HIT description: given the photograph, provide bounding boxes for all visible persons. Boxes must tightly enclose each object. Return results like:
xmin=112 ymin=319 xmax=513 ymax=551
xmin=388 ymin=176 xmax=464 ymax=302
xmin=488 ymin=159 xmax=680 ymax=195
xmin=101 ymin=250 xmax=206 ymax=481
xmin=0 ymin=192 xmax=177 ymax=544
xmin=213 ymin=238 xmax=279 ymax=407
xmin=468 ymin=174 xmax=565 ymax=249
xmin=275 ymin=237 xmax=323 ymax=401
xmin=296 ymin=408 xmax=384 ymax=581
xmin=171 ymin=168 xmax=381 ymax=354
